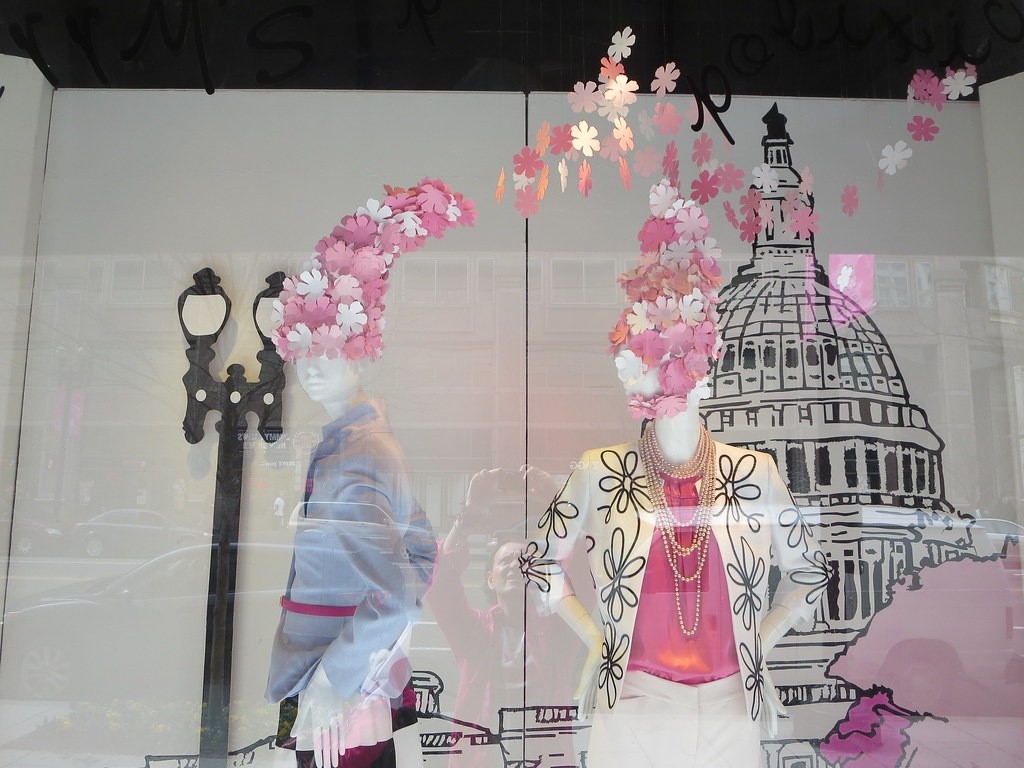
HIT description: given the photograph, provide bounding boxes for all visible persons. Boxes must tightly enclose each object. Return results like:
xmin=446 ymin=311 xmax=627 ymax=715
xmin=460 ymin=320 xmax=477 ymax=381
xmin=543 ymin=327 xmax=834 ymax=768
xmin=262 ymin=353 xmax=440 ymax=768
xmin=427 ymin=462 xmax=596 ymax=768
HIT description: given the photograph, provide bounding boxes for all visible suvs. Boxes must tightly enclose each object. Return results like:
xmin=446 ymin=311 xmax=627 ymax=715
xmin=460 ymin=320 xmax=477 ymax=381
xmin=0 ymin=536 xmax=406 ymax=705
xmin=288 ymin=503 xmax=432 ymax=561
xmin=618 ymin=509 xmax=1024 ymax=714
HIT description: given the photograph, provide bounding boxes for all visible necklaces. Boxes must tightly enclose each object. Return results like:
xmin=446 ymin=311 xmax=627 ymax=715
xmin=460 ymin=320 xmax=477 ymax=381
xmin=641 ymin=420 xmax=717 ymax=635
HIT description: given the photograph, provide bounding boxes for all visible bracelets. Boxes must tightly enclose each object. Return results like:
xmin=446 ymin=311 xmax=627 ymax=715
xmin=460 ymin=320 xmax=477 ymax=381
xmin=453 ymin=504 xmax=474 ymax=543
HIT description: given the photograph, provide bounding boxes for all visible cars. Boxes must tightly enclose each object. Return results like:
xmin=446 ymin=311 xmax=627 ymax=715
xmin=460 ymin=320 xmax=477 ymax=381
xmin=490 ymin=516 xmax=593 ymax=556
xmin=0 ymin=504 xmax=64 ymax=558
xmin=72 ymin=508 xmax=212 ymax=556
xmin=959 ymin=518 xmax=1024 ymax=568
